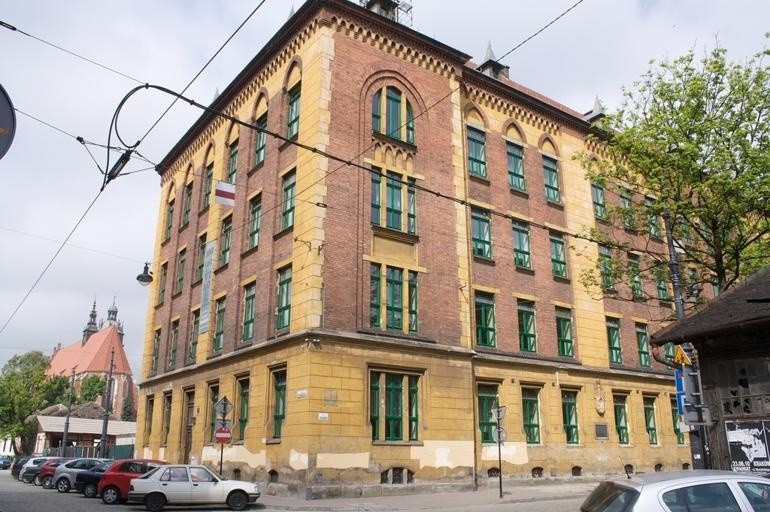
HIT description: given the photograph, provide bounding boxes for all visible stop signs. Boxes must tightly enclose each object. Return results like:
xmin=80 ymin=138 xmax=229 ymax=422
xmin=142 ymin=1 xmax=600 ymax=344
xmin=215 ymin=427 xmax=231 ymax=444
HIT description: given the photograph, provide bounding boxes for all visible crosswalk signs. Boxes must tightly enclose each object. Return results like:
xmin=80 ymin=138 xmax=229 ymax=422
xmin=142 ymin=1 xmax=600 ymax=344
xmin=674 ymin=345 xmax=692 ymax=366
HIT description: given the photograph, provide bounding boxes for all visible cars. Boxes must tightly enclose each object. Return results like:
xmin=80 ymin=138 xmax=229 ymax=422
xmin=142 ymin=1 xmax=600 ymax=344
xmin=580 ymin=469 xmax=770 ymax=512
xmin=0 ymin=458 xmax=261 ymax=511
